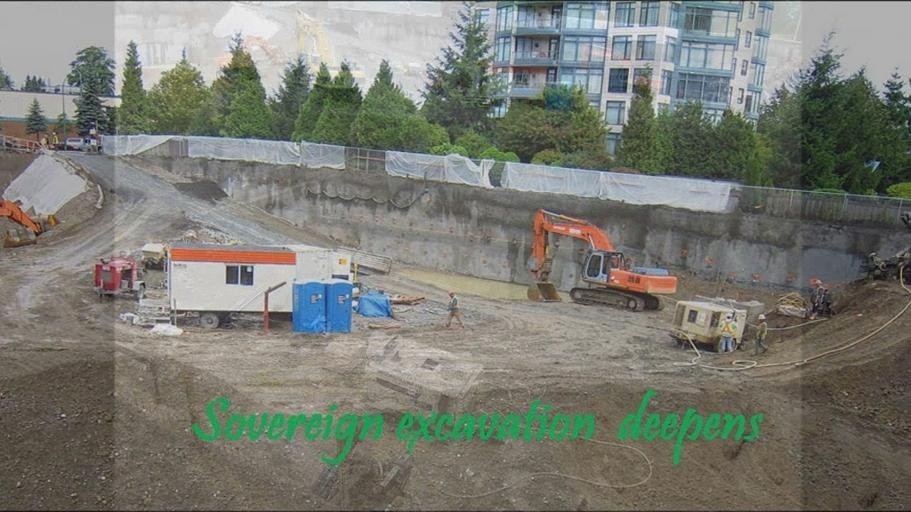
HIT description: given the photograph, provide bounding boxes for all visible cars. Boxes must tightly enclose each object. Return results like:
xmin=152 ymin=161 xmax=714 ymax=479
xmin=67 ymin=138 xmax=84 ymax=150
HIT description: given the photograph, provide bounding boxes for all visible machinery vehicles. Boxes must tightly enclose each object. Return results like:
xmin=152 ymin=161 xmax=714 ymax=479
xmin=527 ymin=209 xmax=678 ymax=312
xmin=0 ymin=199 xmax=56 ymax=246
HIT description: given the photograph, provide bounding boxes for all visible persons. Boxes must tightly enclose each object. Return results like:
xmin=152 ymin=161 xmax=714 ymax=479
xmin=719 ymin=313 xmax=737 ymax=353
xmin=51 ymin=131 xmax=58 ymax=145
xmin=751 ymin=313 xmax=767 ymax=356
xmin=39 ymin=135 xmax=48 ymax=145
xmin=445 ymin=291 xmax=463 ymax=327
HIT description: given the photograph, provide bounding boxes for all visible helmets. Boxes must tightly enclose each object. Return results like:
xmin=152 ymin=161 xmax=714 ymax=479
xmin=815 ymin=280 xmax=823 ymax=285
xmin=727 ymin=314 xmax=733 ymax=317
xmin=758 ymin=313 xmax=767 ymax=320
